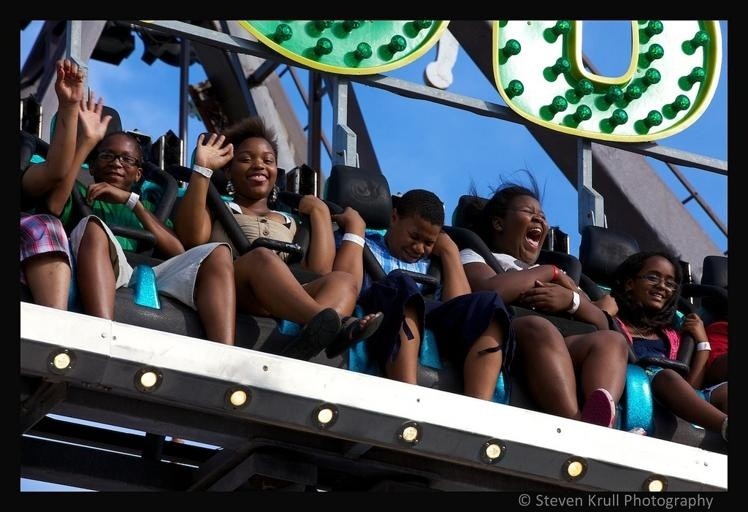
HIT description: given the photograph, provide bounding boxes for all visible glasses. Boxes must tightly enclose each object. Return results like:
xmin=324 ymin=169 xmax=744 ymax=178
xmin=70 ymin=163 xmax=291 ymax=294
xmin=93 ymin=153 xmax=143 ymax=170
xmin=632 ymin=274 xmax=680 ymax=291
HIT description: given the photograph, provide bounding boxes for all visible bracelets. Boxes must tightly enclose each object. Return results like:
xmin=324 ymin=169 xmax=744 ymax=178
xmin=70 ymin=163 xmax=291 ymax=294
xmin=191 ymin=164 xmax=215 ymax=179
xmin=697 ymin=341 xmax=711 ymax=351
xmin=125 ymin=192 xmax=140 ymax=213
xmin=341 ymin=232 xmax=366 ymax=246
xmin=567 ymin=290 xmax=581 ymax=314
xmin=552 ymin=265 xmax=559 ymax=282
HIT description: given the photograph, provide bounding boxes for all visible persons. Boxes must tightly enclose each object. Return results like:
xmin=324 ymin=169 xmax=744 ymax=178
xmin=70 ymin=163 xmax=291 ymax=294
xmin=334 ymin=188 xmax=516 ymax=403
xmin=592 ymin=246 xmax=729 ymax=445
xmin=705 ymin=320 xmax=728 ymax=384
xmin=175 ymin=113 xmax=387 ymax=361
xmin=18 ymin=56 xmax=86 ymax=311
xmin=46 ymin=86 xmax=238 ymax=347
xmin=457 ymin=170 xmax=633 ymax=427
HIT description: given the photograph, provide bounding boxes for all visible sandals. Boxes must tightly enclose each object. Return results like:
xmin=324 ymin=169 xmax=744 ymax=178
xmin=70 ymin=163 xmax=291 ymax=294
xmin=583 ymin=387 xmax=616 ymax=427
xmin=324 ymin=311 xmax=386 ymax=359
xmin=279 ymin=306 xmax=342 ymax=361
